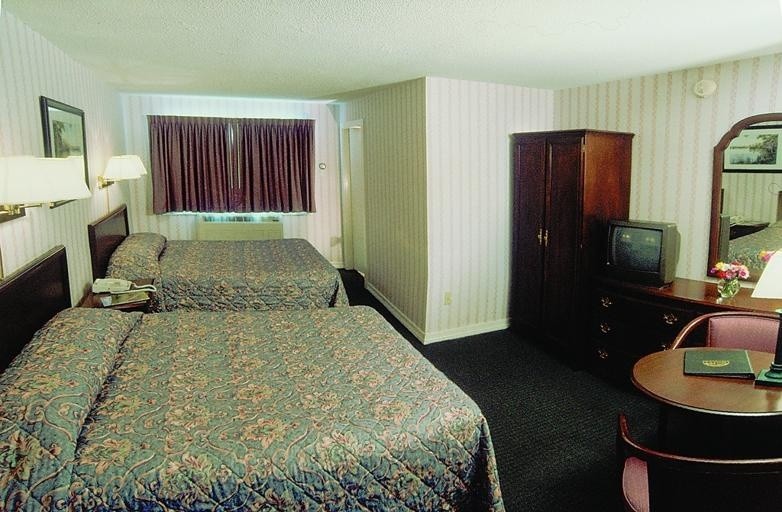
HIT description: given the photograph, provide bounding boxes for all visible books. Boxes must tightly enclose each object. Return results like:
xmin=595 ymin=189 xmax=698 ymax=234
xmin=100 ymin=290 xmax=151 ymax=307
xmin=684 ymin=349 xmax=755 ymax=380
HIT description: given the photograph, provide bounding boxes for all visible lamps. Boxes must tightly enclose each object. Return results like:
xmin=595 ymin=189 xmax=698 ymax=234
xmin=0 ymin=156 xmax=92 ymax=222
xmin=98 ymin=155 xmax=147 ymax=190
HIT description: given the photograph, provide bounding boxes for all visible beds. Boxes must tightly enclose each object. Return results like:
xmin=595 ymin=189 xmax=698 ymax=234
xmin=86 ymin=201 xmax=352 ymax=310
xmin=720 ymin=189 xmax=782 ymax=269
xmin=1 ymin=244 xmax=507 ymax=511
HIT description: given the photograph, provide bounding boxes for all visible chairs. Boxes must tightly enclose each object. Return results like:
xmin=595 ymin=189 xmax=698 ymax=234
xmin=666 ymin=310 xmax=782 ymax=354
xmin=615 ymin=414 xmax=781 ymax=511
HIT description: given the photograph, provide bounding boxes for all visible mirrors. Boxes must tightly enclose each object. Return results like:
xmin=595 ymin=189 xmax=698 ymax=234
xmin=706 ymin=113 xmax=782 ymax=282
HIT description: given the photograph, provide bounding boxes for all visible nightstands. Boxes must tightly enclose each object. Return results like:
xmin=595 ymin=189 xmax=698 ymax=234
xmin=81 ymin=278 xmax=157 ymax=312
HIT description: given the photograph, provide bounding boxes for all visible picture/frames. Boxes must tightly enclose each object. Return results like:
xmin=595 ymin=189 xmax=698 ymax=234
xmin=39 ymin=95 xmax=91 ymax=210
xmin=722 ymin=125 xmax=782 ymax=173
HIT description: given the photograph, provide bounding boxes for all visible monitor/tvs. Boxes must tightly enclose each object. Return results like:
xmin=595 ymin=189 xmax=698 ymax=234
xmin=603 ymin=219 xmax=680 ymax=290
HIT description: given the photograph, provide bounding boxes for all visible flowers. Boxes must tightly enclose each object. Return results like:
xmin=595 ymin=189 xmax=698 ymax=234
xmin=708 ymin=261 xmax=750 ymax=293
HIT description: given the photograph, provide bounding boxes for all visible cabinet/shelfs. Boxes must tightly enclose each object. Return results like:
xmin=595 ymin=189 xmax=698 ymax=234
xmin=508 ymin=128 xmax=635 ymax=348
xmin=584 ymin=276 xmax=781 ymax=388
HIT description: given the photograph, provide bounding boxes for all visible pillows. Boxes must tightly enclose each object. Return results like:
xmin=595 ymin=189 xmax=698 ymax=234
xmin=0 ymin=306 xmax=147 ymax=511
xmin=106 ymin=233 xmax=168 ymax=311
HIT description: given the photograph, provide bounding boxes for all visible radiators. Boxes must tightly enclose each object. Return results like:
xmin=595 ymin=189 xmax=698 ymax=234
xmin=197 ymin=214 xmax=284 ymax=240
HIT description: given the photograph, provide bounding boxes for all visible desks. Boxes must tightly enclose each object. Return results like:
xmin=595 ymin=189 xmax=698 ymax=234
xmin=631 ymin=348 xmax=781 ymax=421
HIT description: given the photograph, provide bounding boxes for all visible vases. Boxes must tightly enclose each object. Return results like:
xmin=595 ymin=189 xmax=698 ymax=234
xmin=717 ymin=278 xmax=741 ymax=299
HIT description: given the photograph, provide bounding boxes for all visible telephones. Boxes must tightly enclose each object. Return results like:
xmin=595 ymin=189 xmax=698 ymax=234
xmin=729 ymin=216 xmax=743 ymax=224
xmin=91 ymin=278 xmax=132 ymax=293
